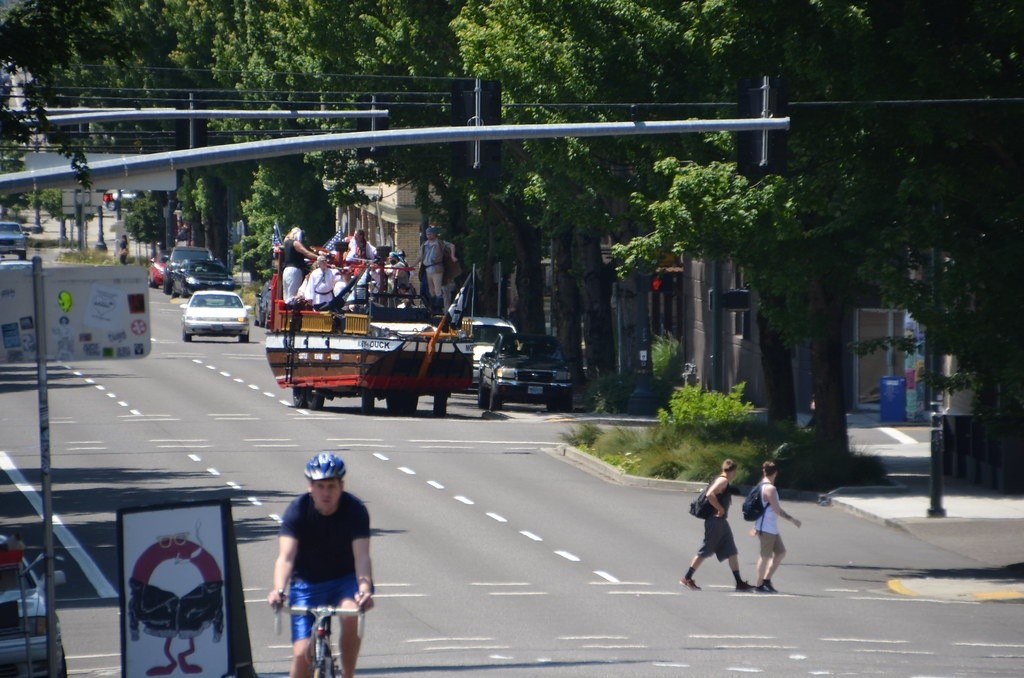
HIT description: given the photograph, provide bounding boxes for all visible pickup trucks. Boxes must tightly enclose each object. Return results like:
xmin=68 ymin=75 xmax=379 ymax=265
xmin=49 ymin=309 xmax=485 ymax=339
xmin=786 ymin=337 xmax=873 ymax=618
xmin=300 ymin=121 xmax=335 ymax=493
xmin=477 ymin=332 xmax=575 ymax=413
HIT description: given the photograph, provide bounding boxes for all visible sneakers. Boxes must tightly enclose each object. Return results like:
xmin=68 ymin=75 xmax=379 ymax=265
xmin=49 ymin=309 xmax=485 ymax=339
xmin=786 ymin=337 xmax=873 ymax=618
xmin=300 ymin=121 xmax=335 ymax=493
xmin=762 ymin=579 xmax=779 ymax=593
xmin=679 ymin=576 xmax=701 ymax=592
xmin=754 ymin=584 xmax=776 ymax=594
xmin=735 ymin=580 xmax=756 ymax=592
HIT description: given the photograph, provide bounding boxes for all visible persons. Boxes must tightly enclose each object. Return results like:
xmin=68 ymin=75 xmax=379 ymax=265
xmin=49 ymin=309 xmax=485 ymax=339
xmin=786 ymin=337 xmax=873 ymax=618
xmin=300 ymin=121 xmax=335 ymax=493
xmin=117 ymin=234 xmax=129 ymax=265
xmin=409 ymin=228 xmax=459 ymax=315
xmin=265 ymin=454 xmax=376 ymax=678
xmin=679 ymin=460 xmax=757 ymax=593
xmin=282 ymin=222 xmax=416 ymax=310
xmin=750 ymin=462 xmax=801 ymax=593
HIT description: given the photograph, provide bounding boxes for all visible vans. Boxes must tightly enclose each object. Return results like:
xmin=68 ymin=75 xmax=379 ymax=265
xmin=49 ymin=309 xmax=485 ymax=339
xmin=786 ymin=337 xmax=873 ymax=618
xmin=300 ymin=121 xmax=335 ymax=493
xmin=461 ymin=320 xmax=520 ymax=385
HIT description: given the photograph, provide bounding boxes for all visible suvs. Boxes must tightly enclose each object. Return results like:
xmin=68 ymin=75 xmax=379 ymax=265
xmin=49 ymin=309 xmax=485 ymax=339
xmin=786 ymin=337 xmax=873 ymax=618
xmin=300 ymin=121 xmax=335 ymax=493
xmin=161 ymin=246 xmax=213 ymax=295
xmin=0 ymin=222 xmax=30 ymax=262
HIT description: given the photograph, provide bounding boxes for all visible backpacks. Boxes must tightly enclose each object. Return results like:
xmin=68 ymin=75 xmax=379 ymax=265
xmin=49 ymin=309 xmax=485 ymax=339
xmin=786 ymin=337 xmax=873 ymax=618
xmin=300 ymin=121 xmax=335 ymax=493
xmin=688 ymin=476 xmax=731 ymax=519
xmin=742 ymin=483 xmax=772 ymax=522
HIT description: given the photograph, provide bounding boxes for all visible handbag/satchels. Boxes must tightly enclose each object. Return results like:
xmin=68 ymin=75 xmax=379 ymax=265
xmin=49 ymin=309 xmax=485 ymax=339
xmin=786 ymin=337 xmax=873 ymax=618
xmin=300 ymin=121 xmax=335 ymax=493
xmin=118 ymin=247 xmax=129 ymax=256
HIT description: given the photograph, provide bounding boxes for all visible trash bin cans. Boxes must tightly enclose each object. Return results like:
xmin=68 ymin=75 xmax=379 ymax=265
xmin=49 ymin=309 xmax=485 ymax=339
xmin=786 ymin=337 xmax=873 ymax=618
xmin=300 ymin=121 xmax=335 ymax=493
xmin=879 ymin=375 xmax=907 ymax=422
xmin=942 ymin=415 xmax=972 ymax=477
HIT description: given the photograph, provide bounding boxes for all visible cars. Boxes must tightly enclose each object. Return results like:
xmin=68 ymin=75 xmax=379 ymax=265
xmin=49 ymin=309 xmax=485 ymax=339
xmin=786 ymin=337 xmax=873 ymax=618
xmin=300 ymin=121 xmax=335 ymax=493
xmin=104 ymin=189 xmax=137 ymax=212
xmin=171 ymin=258 xmax=236 ymax=301
xmin=0 ymin=535 xmax=67 ymax=678
xmin=148 ymin=250 xmax=171 ymax=289
xmin=254 ymin=280 xmax=272 ymax=329
xmin=179 ymin=290 xmax=252 ymax=343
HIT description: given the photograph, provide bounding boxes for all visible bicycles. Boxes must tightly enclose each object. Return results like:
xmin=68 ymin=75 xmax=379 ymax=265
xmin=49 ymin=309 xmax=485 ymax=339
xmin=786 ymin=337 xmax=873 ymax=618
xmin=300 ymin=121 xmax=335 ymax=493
xmin=274 ymin=589 xmax=367 ymax=678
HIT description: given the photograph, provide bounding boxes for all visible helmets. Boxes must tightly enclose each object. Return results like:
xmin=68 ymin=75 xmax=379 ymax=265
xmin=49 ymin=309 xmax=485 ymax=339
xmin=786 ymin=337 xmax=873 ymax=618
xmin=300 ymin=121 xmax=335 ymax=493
xmin=303 ymin=451 xmax=348 ymax=481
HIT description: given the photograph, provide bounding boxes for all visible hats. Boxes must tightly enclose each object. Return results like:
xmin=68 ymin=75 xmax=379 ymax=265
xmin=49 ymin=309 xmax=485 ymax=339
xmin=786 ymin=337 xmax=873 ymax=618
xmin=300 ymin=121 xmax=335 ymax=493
xmin=396 ymin=249 xmax=403 ymax=255
xmin=426 ymin=225 xmax=436 ymax=233
xmin=317 ymin=255 xmax=326 ymax=262
xmin=389 ymin=251 xmax=398 ymax=257
